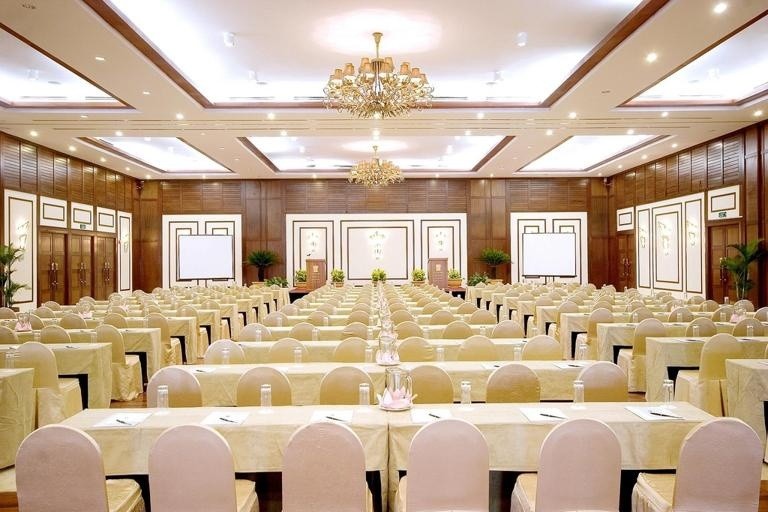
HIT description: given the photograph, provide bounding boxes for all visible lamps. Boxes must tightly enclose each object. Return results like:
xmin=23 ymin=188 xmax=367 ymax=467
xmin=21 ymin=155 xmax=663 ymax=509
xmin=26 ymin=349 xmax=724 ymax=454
xmin=345 ymin=146 xmax=405 ymax=190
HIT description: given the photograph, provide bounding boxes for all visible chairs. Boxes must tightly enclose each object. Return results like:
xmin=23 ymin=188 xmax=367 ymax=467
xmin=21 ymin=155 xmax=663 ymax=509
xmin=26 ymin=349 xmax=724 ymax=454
xmin=322 ymin=32 xmax=434 ymax=119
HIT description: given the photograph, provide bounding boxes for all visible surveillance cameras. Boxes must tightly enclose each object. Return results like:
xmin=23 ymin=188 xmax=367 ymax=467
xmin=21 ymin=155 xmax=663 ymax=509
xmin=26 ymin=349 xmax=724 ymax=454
xmin=604 ymin=177 xmax=611 ymax=185
xmin=137 ymin=180 xmax=144 ymax=186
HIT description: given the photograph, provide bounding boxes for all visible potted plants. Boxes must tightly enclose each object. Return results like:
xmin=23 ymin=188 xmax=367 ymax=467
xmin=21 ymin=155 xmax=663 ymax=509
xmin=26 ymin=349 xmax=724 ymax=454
xmin=330 ymin=269 xmax=345 ymax=287
xmin=412 ymin=269 xmax=426 ymax=285
xmin=295 ymin=270 xmax=307 ymax=288
xmin=480 ymin=248 xmax=512 ymax=279
xmin=447 ymin=269 xmax=463 ymax=286
xmin=372 ymin=271 xmax=387 ymax=286
xmin=243 ymin=249 xmax=278 ymax=286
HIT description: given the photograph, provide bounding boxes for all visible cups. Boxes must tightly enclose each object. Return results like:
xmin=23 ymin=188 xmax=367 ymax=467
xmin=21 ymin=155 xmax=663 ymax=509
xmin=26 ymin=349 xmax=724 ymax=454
xmin=32 ymin=330 xmax=41 ymax=343
xmin=423 ymin=327 xmax=429 ymax=338
xmin=157 ymin=386 xmax=168 ymax=412
xmin=677 ymin=311 xmax=683 ymax=322
xmin=366 ymin=327 xmax=374 ymax=339
xmin=579 ymin=344 xmax=587 ymax=360
xmin=572 ymin=381 xmax=584 ymax=405
xmin=90 ymin=330 xmax=97 ymax=345
xmin=311 ymin=328 xmax=320 ymax=341
xmin=724 ymin=297 xmax=730 ymax=304
xmin=95 ymin=319 xmax=104 ymax=326
xmin=142 ymin=317 xmax=148 ymax=328
xmin=703 ymin=302 xmax=708 ymax=312
xmin=368 ymin=317 xmax=374 ymax=326
xmin=747 ymin=326 xmax=753 ymax=337
xmin=181 ymin=309 xmax=186 ymax=317
xmin=436 ymin=348 xmax=444 ymax=363
xmin=15 ymin=312 xmax=30 ymax=328
xmin=255 ymin=327 xmax=261 ymax=342
xmin=221 ymin=348 xmax=231 ymax=367
xmin=633 ymin=312 xmax=638 ymax=323
xmin=720 ymin=312 xmax=726 ymax=322
xmin=413 ymin=314 xmax=419 ymax=324
xmin=323 ymin=317 xmax=329 ymax=326
xmin=294 ymin=347 xmax=302 ymax=364
xmin=261 ymin=384 xmax=272 ymax=410
xmin=693 ymin=326 xmax=700 ymax=337
xmin=364 ymin=346 xmax=372 ymax=362
xmin=514 ymin=345 xmax=522 ymax=360
xmin=662 ymin=380 xmax=674 ymax=404
xmin=276 ymin=317 xmax=283 ymax=326
xmin=144 ymin=306 xmax=150 ymax=317
xmin=358 ymin=383 xmax=371 ymax=406
xmin=4 ymin=352 xmax=14 ymax=369
xmin=480 ymin=326 xmax=487 ymax=336
xmin=460 ymin=381 xmax=472 ymax=407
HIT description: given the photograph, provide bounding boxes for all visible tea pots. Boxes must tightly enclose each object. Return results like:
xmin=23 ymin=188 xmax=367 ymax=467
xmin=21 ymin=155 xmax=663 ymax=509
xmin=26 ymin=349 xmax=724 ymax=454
xmin=376 ymin=279 xmax=394 ymax=333
xmin=385 ymin=367 xmax=412 ymax=400
xmin=379 ymin=333 xmax=398 ymax=360
